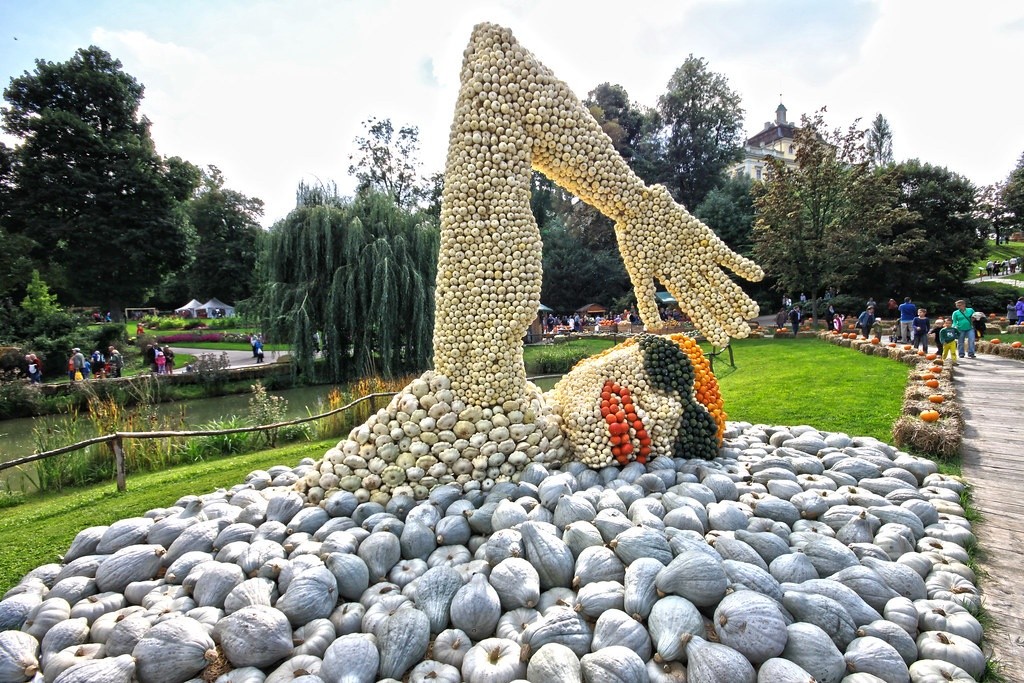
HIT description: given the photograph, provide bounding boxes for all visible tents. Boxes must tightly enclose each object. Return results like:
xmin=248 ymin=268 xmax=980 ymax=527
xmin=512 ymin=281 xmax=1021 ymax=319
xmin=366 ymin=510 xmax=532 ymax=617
xmin=174 ymin=297 xmax=236 ymax=319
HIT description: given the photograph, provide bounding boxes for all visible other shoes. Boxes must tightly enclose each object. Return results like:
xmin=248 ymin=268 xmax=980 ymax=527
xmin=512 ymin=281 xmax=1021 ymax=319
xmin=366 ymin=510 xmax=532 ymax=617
xmin=960 ymin=354 xmax=964 ymax=358
xmin=968 ymin=353 xmax=976 ymax=357
xmin=953 ymin=363 xmax=959 ymax=366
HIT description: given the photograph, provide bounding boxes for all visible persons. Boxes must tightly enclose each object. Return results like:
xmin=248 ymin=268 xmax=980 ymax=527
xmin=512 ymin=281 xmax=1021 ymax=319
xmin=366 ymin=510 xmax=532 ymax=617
xmin=249 ymin=332 xmax=264 ymax=363
xmin=912 ymin=308 xmax=930 ymax=354
xmin=25 ymin=353 xmax=43 ymax=384
xmin=898 ymin=297 xmax=917 ymax=345
xmin=91 ymin=351 xmax=106 ymax=379
xmin=1006 ymin=296 xmax=1024 ymax=325
xmin=285 ymin=21 xmax=765 ymax=504
xmin=108 ymin=346 xmax=124 ymax=378
xmin=952 ymin=300 xmax=976 ymax=359
xmin=986 ymin=256 xmax=1024 ymax=275
xmin=940 ymin=317 xmax=961 ymax=367
xmin=542 ymin=307 xmax=681 ymax=335
xmin=67 ymin=347 xmax=91 ymax=381
xmin=825 ymin=304 xmax=844 ymax=333
xmin=928 ymin=318 xmax=945 ymax=355
xmin=147 ymin=343 xmax=175 ymax=374
xmin=137 ymin=323 xmax=144 ymax=338
xmin=888 ymin=299 xmax=898 ymax=320
xmin=858 ymin=297 xmax=884 ymax=342
xmin=775 ymin=293 xmax=807 ymax=334
xmin=825 ymin=286 xmax=835 ymax=299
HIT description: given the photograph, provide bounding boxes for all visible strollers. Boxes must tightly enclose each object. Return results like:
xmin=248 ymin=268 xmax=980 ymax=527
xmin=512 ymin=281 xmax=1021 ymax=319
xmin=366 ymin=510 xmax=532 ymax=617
xmin=889 ymin=317 xmax=911 ymax=343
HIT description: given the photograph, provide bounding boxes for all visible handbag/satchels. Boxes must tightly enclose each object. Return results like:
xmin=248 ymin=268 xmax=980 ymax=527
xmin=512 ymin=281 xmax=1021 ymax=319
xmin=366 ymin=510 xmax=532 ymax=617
xmin=975 ymin=329 xmax=981 ymax=338
xmin=75 ymin=370 xmax=83 ymax=382
xmin=258 ymin=348 xmax=263 ymax=354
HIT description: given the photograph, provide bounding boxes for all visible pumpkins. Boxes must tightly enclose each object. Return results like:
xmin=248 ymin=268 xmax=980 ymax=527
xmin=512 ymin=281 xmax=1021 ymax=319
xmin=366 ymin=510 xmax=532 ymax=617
xmin=598 ymin=319 xmax=616 ymax=326
xmin=990 ymin=313 xmax=995 ymax=317
xmin=752 ymin=326 xmax=762 ymax=334
xmin=776 ymin=317 xmax=855 ymax=334
xmin=1021 ymin=321 xmax=1024 ymax=325
xmin=841 ymin=332 xmax=944 ymax=422
xmin=990 ymin=338 xmax=1000 ymax=344
xmin=1011 ymin=341 xmax=1022 ymax=348
xmin=1000 ymin=317 xmax=1005 ymax=321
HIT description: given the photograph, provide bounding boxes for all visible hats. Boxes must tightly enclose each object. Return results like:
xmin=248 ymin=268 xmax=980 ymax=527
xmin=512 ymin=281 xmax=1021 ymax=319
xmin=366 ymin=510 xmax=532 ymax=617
xmin=159 ymin=352 xmax=164 ymax=355
xmin=72 ymin=348 xmax=80 ymax=351
xmin=165 ymin=345 xmax=169 ymax=348
xmin=95 ymin=351 xmax=99 ymax=354
xmin=875 ymin=317 xmax=881 ymax=322
